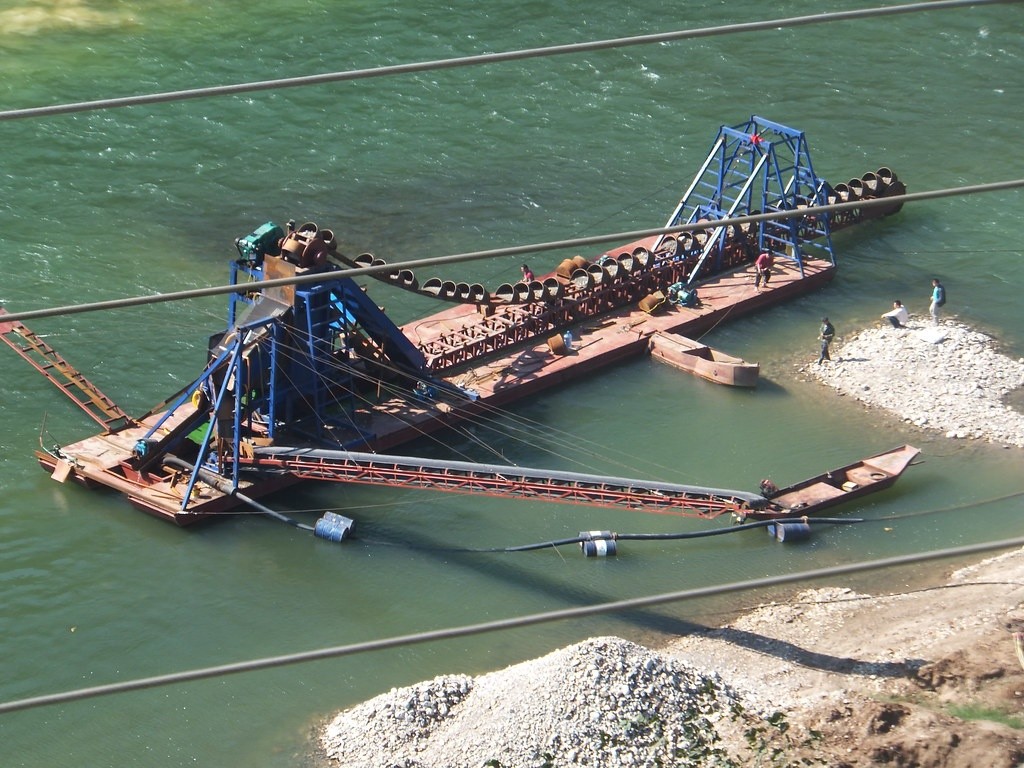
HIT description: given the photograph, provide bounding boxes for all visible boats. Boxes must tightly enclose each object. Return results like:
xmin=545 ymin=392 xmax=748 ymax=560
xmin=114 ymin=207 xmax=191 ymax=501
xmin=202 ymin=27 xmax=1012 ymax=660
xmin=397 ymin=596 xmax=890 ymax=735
xmin=647 ymin=328 xmax=760 ymax=388
xmin=736 ymin=443 xmax=922 ymax=524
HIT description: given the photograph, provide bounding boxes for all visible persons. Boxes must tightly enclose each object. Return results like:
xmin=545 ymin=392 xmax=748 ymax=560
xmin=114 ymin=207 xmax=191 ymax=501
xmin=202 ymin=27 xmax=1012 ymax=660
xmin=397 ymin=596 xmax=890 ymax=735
xmin=881 ymin=299 xmax=908 ymax=328
xmin=818 ymin=316 xmax=835 ymax=364
xmin=754 ymin=251 xmax=774 ymax=291
xmin=516 ymin=265 xmax=535 ymax=283
xmin=929 ymin=279 xmax=945 ymax=326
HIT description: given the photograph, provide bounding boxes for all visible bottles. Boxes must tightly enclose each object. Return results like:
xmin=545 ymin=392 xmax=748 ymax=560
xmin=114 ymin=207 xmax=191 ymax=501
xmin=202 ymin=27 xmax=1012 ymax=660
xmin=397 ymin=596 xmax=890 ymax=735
xmin=563 ymin=331 xmax=572 ymax=348
